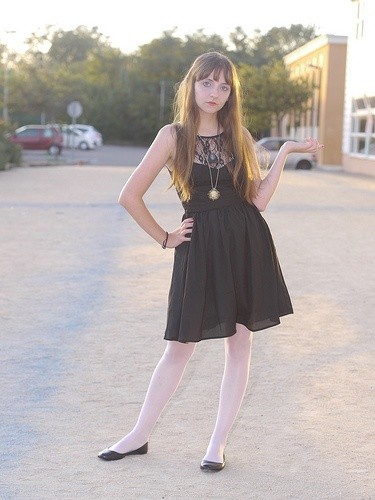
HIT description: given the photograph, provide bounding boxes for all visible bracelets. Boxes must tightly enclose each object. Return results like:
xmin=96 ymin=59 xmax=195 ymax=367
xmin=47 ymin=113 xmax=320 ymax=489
xmin=162 ymin=232 xmax=168 ymax=249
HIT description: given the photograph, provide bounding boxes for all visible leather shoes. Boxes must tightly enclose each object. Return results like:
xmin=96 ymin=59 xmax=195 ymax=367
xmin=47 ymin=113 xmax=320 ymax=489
xmin=200 ymin=452 xmax=227 ymax=472
xmin=97 ymin=441 xmax=149 ymax=461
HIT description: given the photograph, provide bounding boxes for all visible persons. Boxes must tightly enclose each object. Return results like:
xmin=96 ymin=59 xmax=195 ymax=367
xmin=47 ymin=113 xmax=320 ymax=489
xmin=98 ymin=52 xmax=325 ymax=471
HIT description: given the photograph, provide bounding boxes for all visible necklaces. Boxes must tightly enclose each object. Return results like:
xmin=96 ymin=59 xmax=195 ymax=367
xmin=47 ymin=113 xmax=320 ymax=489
xmin=197 ymin=120 xmax=221 ymax=201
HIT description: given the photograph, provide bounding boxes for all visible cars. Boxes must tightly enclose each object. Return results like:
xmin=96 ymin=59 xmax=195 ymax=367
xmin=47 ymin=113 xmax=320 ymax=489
xmin=255 ymin=136 xmax=318 ymax=169
xmin=6 ymin=124 xmax=62 ymax=156
xmin=62 ymin=124 xmax=103 ymax=149
